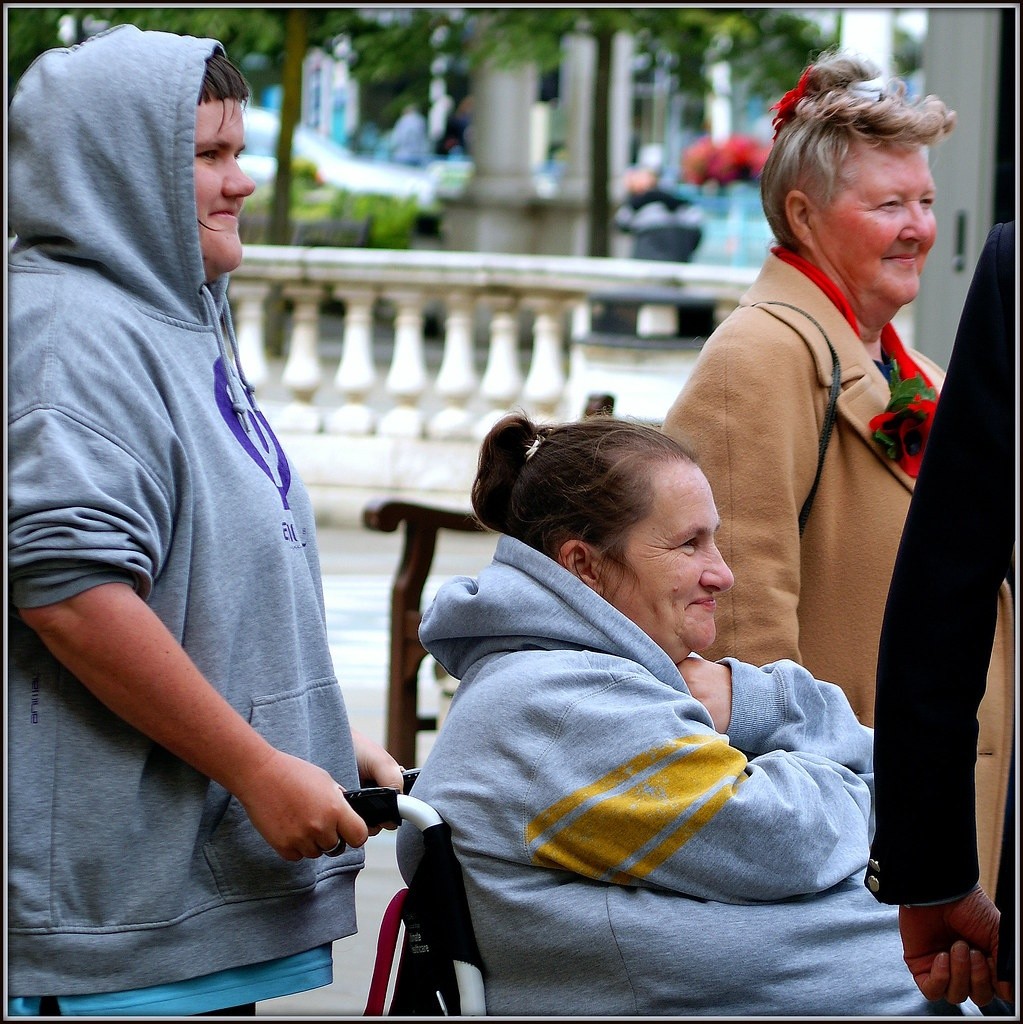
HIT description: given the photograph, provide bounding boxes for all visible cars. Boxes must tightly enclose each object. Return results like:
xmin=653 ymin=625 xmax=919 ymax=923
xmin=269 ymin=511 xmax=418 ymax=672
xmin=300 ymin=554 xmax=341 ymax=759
xmin=233 ymin=103 xmax=444 ymax=225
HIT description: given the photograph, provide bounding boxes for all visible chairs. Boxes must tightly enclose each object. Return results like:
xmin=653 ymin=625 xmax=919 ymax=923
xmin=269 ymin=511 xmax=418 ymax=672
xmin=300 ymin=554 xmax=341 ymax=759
xmin=363 ymin=393 xmax=616 ymax=774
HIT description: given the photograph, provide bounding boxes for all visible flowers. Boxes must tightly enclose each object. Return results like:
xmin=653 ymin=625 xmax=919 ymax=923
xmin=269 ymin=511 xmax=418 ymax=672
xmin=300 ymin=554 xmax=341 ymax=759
xmin=768 ymin=65 xmax=814 ymax=142
xmin=869 ymin=354 xmax=940 ymax=478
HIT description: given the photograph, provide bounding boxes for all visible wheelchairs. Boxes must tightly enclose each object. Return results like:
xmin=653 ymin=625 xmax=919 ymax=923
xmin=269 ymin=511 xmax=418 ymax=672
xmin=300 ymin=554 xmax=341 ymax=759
xmin=339 ymin=764 xmax=1010 ymax=1018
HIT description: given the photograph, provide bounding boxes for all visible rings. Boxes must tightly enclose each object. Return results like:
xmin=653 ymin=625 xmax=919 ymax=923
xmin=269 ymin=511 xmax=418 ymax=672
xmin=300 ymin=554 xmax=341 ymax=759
xmin=322 ymin=838 xmax=346 ymax=857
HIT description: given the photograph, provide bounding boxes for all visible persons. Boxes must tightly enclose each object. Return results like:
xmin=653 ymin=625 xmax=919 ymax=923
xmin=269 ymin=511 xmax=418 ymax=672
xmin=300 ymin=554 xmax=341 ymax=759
xmin=394 ymin=414 xmax=989 ymax=1018
xmin=863 ymin=219 xmax=1016 ymax=1018
xmin=7 ymin=24 xmax=407 ymax=1014
xmin=661 ymin=52 xmax=1012 ymax=911
